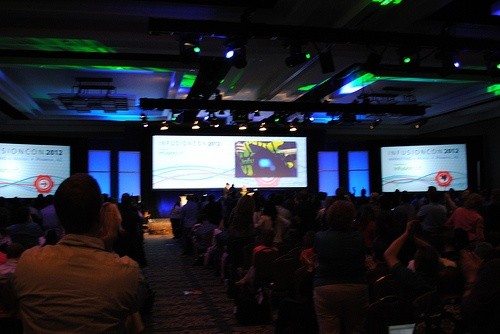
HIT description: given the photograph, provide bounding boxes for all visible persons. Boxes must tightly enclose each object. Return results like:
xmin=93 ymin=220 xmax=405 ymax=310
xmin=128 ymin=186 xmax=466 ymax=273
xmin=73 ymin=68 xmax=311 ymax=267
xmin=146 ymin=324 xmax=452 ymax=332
xmin=0 ymin=181 xmax=500 ymax=334
xmin=14 ymin=172 xmax=147 ymax=334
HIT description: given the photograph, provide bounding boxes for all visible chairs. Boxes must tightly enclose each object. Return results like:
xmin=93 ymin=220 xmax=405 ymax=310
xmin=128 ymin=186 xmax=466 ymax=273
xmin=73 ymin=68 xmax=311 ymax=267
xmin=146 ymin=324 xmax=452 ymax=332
xmin=368 ymin=274 xmax=444 ymax=334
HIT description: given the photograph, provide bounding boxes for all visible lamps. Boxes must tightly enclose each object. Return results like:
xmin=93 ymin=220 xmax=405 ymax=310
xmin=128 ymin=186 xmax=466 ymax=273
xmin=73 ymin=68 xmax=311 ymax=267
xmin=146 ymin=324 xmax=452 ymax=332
xmin=190 ymin=121 xmax=201 ymax=130
xmin=257 ymin=123 xmax=269 ymax=132
xmin=141 ymin=117 xmax=150 ymax=128
xmin=193 ymin=34 xmax=234 ymax=61
xmin=160 ymin=121 xmax=171 ymax=132
xmin=289 ymin=122 xmax=300 ymax=133
xmin=237 ymin=122 xmax=248 ymax=130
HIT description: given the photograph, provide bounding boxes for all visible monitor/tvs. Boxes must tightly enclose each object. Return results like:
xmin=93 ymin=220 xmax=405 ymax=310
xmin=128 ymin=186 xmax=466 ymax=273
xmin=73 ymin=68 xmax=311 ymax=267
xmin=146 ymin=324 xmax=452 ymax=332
xmin=386 ymin=322 xmax=416 ymax=334
